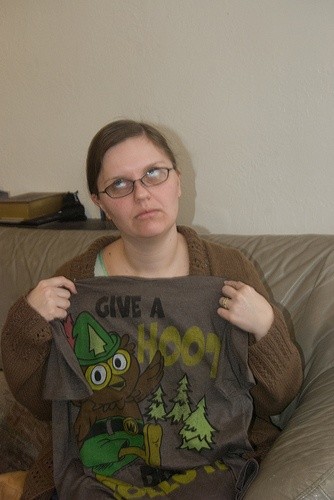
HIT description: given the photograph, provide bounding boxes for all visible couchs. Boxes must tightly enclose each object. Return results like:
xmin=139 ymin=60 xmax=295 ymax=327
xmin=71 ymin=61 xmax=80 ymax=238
xmin=0 ymin=223 xmax=334 ymax=500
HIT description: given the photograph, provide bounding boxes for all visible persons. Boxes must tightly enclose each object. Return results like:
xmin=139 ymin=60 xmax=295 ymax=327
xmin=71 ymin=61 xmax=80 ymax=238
xmin=0 ymin=119 xmax=303 ymax=500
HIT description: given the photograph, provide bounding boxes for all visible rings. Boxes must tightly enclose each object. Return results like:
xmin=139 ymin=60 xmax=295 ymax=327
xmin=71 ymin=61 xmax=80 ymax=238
xmin=222 ymin=298 xmax=228 ymax=309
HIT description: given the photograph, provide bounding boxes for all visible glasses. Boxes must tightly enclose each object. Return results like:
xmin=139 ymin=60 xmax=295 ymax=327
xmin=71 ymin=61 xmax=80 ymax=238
xmin=97 ymin=167 xmax=174 ymax=199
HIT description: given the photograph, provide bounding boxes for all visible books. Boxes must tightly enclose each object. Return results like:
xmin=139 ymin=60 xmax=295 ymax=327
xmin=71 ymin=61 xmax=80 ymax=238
xmin=0 ymin=191 xmax=67 ymax=220
